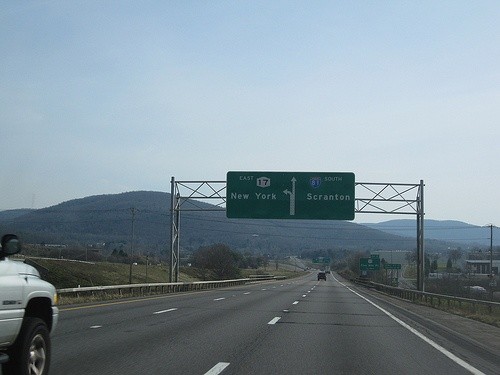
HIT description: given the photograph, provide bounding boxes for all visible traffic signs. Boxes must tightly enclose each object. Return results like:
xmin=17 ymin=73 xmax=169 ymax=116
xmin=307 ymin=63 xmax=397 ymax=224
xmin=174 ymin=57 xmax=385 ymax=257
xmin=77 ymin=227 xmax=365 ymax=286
xmin=226 ymin=170 xmax=356 ymax=221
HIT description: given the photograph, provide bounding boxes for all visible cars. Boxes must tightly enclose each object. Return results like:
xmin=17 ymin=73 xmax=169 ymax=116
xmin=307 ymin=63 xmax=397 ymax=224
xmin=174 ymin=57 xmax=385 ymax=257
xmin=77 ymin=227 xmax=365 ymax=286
xmin=325 ymin=270 xmax=330 ymax=274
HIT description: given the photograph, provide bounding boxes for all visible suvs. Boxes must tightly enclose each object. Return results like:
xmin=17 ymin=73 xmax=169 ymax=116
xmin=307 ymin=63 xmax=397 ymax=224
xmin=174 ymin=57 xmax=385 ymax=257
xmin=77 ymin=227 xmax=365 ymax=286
xmin=0 ymin=232 xmax=60 ymax=375
xmin=317 ymin=272 xmax=326 ymax=281
